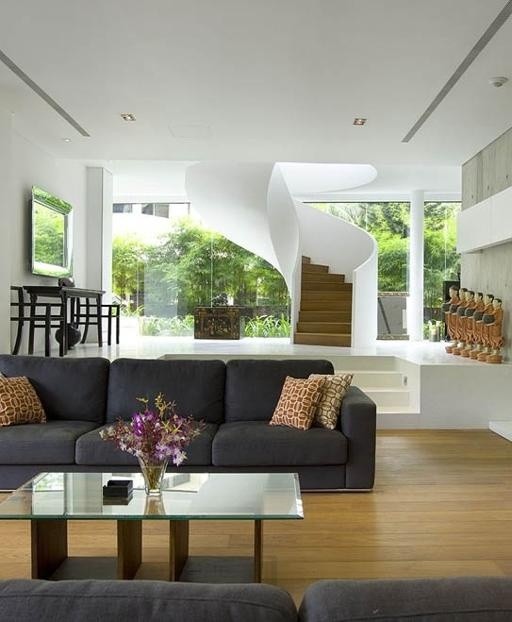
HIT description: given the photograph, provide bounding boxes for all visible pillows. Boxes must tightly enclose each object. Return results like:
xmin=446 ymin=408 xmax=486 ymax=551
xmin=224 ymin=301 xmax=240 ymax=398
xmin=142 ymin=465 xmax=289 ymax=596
xmin=269 ymin=366 xmax=353 ymax=433
xmin=0 ymin=374 xmax=49 ymax=428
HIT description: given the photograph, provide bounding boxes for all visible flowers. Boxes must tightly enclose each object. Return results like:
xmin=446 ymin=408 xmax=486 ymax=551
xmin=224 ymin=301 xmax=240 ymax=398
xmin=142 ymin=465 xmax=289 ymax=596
xmin=97 ymin=390 xmax=211 ymax=484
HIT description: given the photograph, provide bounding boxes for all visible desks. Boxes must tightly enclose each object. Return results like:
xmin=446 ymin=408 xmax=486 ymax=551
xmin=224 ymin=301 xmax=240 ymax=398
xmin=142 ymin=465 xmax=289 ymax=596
xmin=23 ymin=285 xmax=106 ymax=358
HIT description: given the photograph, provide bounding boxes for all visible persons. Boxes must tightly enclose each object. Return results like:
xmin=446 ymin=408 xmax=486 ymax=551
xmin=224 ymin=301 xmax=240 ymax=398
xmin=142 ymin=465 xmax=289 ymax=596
xmin=441 ymin=285 xmax=503 ymax=363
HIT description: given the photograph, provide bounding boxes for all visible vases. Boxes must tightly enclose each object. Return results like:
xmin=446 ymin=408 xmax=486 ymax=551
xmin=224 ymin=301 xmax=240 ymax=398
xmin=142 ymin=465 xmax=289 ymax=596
xmin=56 ymin=322 xmax=81 ymax=350
xmin=136 ymin=454 xmax=171 ymax=490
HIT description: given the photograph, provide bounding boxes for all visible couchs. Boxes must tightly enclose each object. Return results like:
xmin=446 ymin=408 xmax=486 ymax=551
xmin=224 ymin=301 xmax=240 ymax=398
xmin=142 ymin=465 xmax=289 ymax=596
xmin=0 ymin=352 xmax=377 ymax=493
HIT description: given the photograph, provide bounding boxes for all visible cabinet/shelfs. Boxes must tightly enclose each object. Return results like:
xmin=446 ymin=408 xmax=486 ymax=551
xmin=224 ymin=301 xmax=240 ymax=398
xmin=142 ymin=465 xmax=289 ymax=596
xmin=192 ymin=306 xmax=245 ymax=340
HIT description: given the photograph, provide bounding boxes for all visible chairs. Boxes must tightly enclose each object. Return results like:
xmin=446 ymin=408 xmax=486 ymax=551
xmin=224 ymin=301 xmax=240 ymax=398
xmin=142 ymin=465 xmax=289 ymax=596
xmin=71 ymin=294 xmax=121 ymax=345
xmin=298 ymin=573 xmax=509 ymax=622
xmin=0 ymin=573 xmax=298 ymax=622
xmin=11 ymin=285 xmax=65 ymax=357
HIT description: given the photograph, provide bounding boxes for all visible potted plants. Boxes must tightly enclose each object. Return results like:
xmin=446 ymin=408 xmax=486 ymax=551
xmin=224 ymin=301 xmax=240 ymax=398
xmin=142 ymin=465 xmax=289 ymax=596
xmin=57 ymin=270 xmax=74 ymax=286
